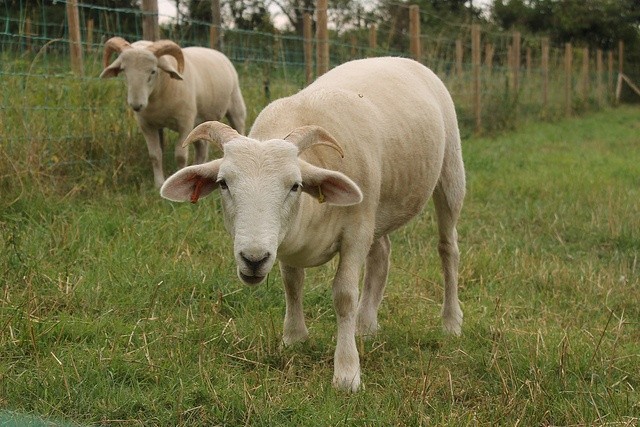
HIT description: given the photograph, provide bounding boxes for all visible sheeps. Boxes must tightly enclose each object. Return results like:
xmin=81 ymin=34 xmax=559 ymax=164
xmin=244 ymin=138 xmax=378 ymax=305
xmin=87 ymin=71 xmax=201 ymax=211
xmin=99 ymin=36 xmax=247 ymax=188
xmin=160 ymin=55 xmax=466 ymax=393
